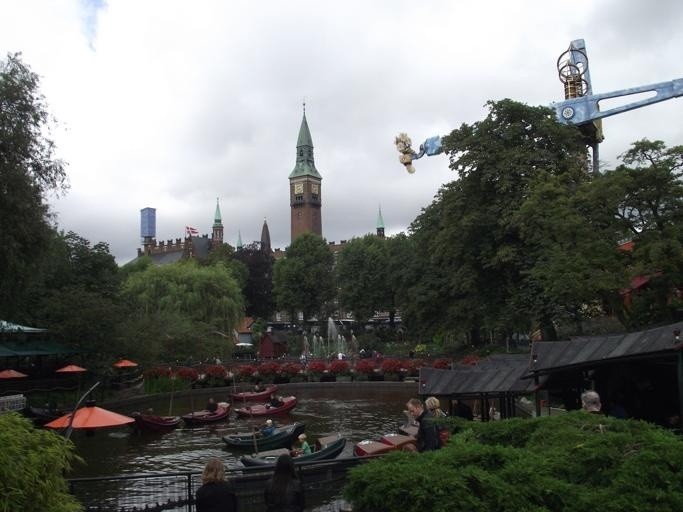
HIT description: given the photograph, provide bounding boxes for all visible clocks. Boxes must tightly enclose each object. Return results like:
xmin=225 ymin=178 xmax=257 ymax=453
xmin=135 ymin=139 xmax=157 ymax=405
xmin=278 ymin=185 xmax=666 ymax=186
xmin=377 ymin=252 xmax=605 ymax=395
xmin=562 ymin=106 xmax=575 ymax=120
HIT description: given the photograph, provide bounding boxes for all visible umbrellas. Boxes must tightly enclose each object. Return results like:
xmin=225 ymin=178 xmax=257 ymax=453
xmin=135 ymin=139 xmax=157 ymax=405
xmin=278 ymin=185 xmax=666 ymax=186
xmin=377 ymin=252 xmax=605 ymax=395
xmin=113 ymin=358 xmax=140 ymax=370
xmin=56 ymin=364 xmax=88 ymax=375
xmin=0 ymin=368 xmax=29 ymax=381
xmin=42 ymin=397 xmax=137 ymax=430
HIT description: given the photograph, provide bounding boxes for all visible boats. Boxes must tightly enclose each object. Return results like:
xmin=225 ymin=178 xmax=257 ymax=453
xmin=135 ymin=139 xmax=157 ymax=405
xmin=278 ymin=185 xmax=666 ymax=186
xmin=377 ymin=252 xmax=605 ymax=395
xmin=381 ymin=433 xmax=415 ymax=452
xmin=228 ymin=382 xmax=279 ymax=401
xmin=397 ymin=423 xmax=419 ymax=438
xmin=221 ymin=420 xmax=305 ymax=448
xmin=233 ymin=394 xmax=297 ymax=416
xmin=239 ymin=433 xmax=346 ymax=470
xmin=125 ymin=409 xmax=180 ymax=430
xmin=352 ymin=438 xmax=394 ymax=457
xmin=181 ymin=400 xmax=231 ymax=423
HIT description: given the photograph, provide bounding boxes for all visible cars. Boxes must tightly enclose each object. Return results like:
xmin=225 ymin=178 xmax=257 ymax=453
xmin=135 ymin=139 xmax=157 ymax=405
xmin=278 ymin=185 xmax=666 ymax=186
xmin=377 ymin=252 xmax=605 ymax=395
xmin=186 ymin=371 xmax=233 ymax=390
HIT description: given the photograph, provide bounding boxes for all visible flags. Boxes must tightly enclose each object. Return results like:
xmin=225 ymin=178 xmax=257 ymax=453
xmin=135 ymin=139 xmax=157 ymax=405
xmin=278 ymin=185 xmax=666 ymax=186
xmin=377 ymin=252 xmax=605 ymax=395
xmin=185 ymin=225 xmax=201 ymax=236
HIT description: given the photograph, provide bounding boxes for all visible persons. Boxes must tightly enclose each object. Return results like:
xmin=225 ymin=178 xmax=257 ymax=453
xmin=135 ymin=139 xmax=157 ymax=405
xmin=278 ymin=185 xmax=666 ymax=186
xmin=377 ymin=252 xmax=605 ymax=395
xmin=424 ymin=395 xmax=450 ymax=448
xmin=550 ymin=376 xmax=629 ymax=419
xmin=145 ymin=407 xmax=153 ymax=415
xmin=404 ymin=398 xmax=440 ymax=453
xmin=337 ymin=350 xmax=346 ymax=360
xmin=274 ymin=396 xmax=284 ymax=407
xmin=294 ymin=433 xmax=311 ymax=457
xmin=206 ymin=398 xmax=217 ymax=412
xmin=451 ymin=398 xmax=473 ymax=421
xmin=262 ymin=454 xmax=306 ymax=512
xmin=270 ymin=393 xmax=276 ymax=404
xmin=255 ymin=381 xmax=266 ymax=393
xmin=259 ymin=418 xmax=275 ymax=437
xmin=194 ymin=459 xmax=240 ymax=512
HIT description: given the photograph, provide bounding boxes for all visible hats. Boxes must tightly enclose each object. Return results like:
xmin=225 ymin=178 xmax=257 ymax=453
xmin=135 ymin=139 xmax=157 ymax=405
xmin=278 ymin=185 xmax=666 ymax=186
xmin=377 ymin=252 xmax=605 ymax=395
xmin=581 ymin=391 xmax=601 ymax=412
